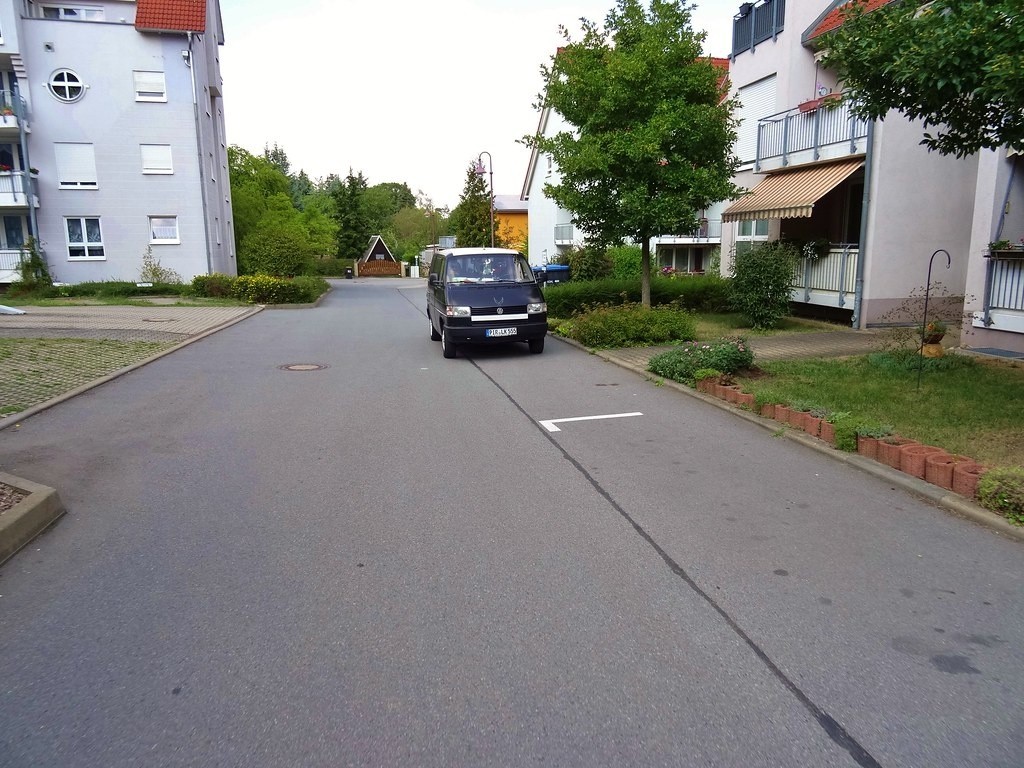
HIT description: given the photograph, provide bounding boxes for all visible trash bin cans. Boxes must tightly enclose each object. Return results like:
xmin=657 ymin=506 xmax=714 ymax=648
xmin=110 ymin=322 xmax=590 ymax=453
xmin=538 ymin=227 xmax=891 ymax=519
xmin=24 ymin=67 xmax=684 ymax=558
xmin=346 ymin=267 xmax=352 ymax=279
xmin=546 ymin=264 xmax=570 ymax=286
xmin=531 ymin=266 xmax=547 ymax=283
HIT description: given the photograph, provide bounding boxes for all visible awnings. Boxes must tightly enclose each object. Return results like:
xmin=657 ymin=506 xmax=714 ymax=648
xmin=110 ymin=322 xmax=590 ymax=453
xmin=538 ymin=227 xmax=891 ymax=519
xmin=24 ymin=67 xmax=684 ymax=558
xmin=721 ymin=156 xmax=866 ymax=223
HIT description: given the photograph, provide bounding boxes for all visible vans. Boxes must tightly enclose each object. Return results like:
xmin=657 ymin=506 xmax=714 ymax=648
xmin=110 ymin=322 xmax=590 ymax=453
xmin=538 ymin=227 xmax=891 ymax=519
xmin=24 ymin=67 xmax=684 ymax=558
xmin=425 ymin=246 xmax=549 ymax=358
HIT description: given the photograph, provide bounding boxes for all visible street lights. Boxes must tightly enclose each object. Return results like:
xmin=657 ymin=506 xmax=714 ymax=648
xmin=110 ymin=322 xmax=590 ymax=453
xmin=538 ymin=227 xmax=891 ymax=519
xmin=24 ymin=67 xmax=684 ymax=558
xmin=474 ymin=151 xmax=495 ymax=248
